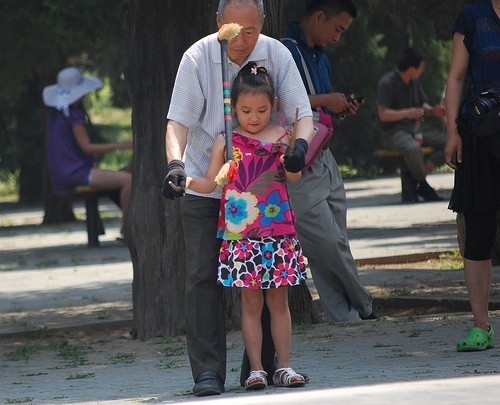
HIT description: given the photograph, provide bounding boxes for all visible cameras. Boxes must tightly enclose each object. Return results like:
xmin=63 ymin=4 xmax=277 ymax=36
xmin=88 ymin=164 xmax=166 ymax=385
xmin=470 ymin=87 xmax=500 ymax=118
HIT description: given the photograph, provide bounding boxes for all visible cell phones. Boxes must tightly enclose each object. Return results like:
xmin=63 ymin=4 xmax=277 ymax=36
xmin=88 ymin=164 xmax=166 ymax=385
xmin=346 ymin=94 xmax=355 ymax=102
xmin=356 ymin=96 xmax=364 ymax=102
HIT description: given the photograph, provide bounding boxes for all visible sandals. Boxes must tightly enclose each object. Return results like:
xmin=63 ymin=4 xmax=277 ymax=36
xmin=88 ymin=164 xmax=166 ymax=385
xmin=243 ymin=370 xmax=269 ymax=390
xmin=455 ymin=326 xmax=497 ymax=351
xmin=272 ymin=367 xmax=306 ymax=387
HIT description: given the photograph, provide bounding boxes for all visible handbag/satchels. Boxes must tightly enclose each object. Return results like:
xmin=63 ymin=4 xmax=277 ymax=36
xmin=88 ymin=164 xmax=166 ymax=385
xmin=279 ymin=36 xmax=333 ymax=173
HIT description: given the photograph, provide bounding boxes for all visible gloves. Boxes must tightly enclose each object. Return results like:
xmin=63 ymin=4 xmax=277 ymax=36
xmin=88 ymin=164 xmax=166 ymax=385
xmin=162 ymin=160 xmax=187 ymax=200
xmin=282 ymin=138 xmax=309 ymax=173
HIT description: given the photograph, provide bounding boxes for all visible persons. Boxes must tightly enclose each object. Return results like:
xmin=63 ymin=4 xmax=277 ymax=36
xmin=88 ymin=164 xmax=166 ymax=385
xmin=185 ymin=60 xmax=306 ymax=389
xmin=279 ymin=0 xmax=377 ymax=324
xmin=377 ymin=0 xmax=500 ymax=351
xmin=162 ymin=0 xmax=315 ymax=397
xmin=42 ymin=66 xmax=135 ymax=243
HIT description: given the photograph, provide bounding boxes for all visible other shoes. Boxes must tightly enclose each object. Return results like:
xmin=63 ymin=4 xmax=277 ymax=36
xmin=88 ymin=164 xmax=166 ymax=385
xmin=240 ymin=367 xmax=310 ymax=387
xmin=401 ymin=193 xmax=420 ymax=203
xmin=192 ymin=371 xmax=225 ymax=397
xmin=419 ymin=187 xmax=444 ymax=201
xmin=363 ymin=311 xmax=377 ymax=321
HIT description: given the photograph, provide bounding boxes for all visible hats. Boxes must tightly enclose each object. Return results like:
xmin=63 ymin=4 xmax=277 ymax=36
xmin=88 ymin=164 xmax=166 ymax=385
xmin=41 ymin=67 xmax=103 ymax=119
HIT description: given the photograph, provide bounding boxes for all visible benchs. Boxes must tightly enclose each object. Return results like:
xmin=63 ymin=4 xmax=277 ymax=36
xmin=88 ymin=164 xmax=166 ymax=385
xmin=374 ymin=144 xmax=432 ymax=205
xmin=74 ymin=184 xmax=122 ymax=248
